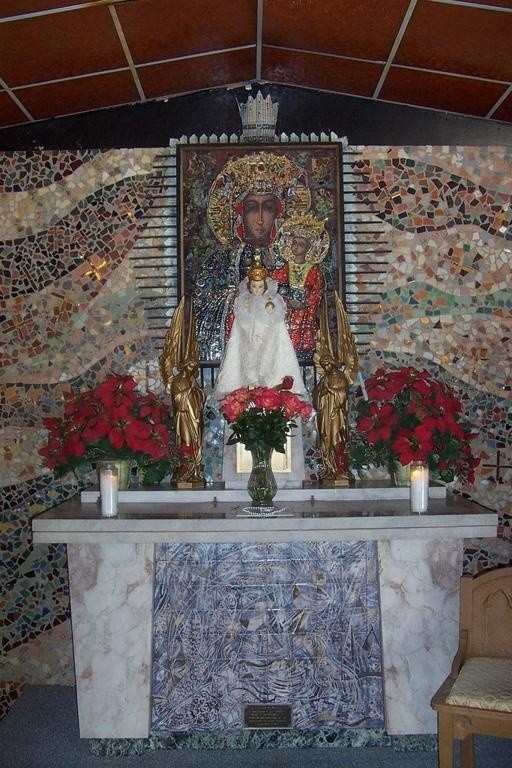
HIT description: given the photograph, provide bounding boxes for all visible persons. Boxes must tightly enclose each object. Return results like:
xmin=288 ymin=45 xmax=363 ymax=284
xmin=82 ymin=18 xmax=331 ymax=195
xmin=313 ymin=353 xmax=353 ymax=480
xmin=274 ymin=232 xmax=321 ymax=357
xmin=169 ymin=355 xmax=203 ymax=482
xmin=192 ymin=187 xmax=283 ymax=361
xmin=220 ymin=261 xmax=298 ymax=396
xmin=263 ymin=296 xmax=277 ymax=315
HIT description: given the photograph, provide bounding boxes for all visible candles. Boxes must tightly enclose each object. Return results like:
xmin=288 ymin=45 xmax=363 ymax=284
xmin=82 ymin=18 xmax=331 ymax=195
xmin=411 ymin=468 xmax=429 ymax=511
xmin=100 ymin=473 xmax=118 ymax=516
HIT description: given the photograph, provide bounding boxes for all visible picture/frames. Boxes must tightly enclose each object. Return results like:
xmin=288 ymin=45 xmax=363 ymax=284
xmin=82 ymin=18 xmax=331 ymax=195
xmin=176 ymin=140 xmax=347 ymax=368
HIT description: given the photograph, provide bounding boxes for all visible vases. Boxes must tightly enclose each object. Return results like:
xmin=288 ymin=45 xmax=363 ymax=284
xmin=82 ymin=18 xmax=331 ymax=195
xmin=90 ymin=456 xmax=134 ymax=490
xmin=246 ymin=446 xmax=278 ymax=507
xmin=391 ymin=460 xmax=429 ymax=487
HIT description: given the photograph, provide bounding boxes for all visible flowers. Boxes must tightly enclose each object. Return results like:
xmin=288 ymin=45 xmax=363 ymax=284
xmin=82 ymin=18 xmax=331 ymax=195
xmin=337 ymin=367 xmax=481 ymax=485
xmin=39 ymin=373 xmax=194 ymax=486
xmin=217 ymin=382 xmax=312 ymax=499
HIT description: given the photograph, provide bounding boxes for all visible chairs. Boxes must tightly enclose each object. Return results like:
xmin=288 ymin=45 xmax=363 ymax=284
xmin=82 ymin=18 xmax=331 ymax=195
xmin=431 ymin=566 xmax=512 ymax=766
